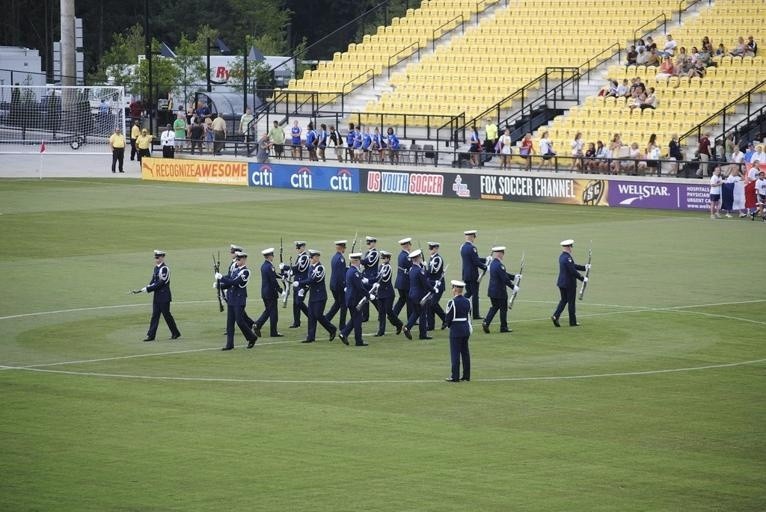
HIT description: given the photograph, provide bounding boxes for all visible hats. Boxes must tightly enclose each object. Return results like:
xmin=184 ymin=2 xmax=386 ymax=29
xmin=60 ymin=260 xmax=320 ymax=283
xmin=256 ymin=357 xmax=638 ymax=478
xmin=463 ymin=230 xmax=476 ymax=234
xmin=492 ymin=246 xmax=506 ymax=251
xmin=153 ymin=249 xmax=167 ymax=257
xmin=230 ymin=241 xmax=321 ymax=258
xmin=560 ymin=238 xmax=575 ymax=248
xmin=334 ymin=237 xmax=439 ymax=260
xmin=451 ymin=279 xmax=465 ymax=289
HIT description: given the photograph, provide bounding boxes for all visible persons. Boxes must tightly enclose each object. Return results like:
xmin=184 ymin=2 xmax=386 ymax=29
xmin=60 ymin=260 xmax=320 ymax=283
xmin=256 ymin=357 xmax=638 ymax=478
xmin=239 ymin=107 xmax=255 ymax=144
xmin=470 ymin=119 xmax=766 ymax=221
xmin=256 ymin=120 xmax=399 ymax=164
xmin=363 ymin=251 xmax=403 ymax=336
xmin=359 ymin=236 xmax=380 ymax=321
xmin=444 ymin=280 xmax=472 ymax=381
xmin=98 ymin=96 xmax=228 ymax=173
xmin=551 ymin=239 xmax=591 ymax=327
xmin=279 ymin=241 xmax=310 ymax=327
xmin=422 ymin=242 xmax=449 ymax=331
xmin=324 ymin=240 xmax=348 ymax=330
xmin=390 ymin=238 xmax=419 ymax=324
xmin=143 ymin=250 xmax=181 ymax=342
xmin=292 ymin=250 xmax=337 ymax=343
xmin=215 ymin=245 xmax=257 ymax=350
xmin=404 ymin=250 xmax=434 ymax=340
xmin=459 ymin=231 xmax=491 ymax=319
xmin=599 ymin=78 xmax=658 ymax=113
xmin=627 ymin=33 xmax=757 ymax=77
xmin=338 ymin=253 xmax=369 ymax=346
xmin=255 ymin=247 xmax=284 ymax=337
xmin=481 ymin=247 xmax=520 ymax=333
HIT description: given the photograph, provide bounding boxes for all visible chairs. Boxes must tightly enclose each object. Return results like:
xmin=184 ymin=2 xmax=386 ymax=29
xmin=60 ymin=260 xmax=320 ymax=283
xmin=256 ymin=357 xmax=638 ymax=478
xmin=266 ymin=0 xmax=766 ymax=167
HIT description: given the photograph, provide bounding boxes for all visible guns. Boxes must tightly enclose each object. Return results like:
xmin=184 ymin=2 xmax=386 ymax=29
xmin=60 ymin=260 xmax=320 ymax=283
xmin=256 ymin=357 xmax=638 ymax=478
xmin=477 ymin=246 xmax=496 ymax=283
xmin=348 ymin=233 xmax=358 ymax=267
xmin=419 ymin=242 xmax=427 ymax=272
xmin=281 ymin=256 xmax=292 ymax=307
xmin=579 ymin=249 xmax=591 ymax=300
xmin=509 ymin=251 xmax=525 ymax=310
xmin=286 ymin=278 xmax=312 ymax=289
xmin=127 ymin=291 xmax=143 ymax=294
xmin=213 ymin=255 xmax=228 ymax=303
xmin=419 ymin=265 xmax=448 ymax=305
xmin=280 ymin=235 xmax=283 ymax=277
xmin=355 ymin=268 xmax=386 ymax=310
xmin=216 ymin=251 xmax=223 ymax=313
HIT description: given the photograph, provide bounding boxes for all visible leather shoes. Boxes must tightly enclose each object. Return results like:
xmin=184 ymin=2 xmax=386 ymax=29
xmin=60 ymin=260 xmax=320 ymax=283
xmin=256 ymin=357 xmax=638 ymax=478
xmin=142 ymin=335 xmax=154 ymax=341
xmin=221 ymin=323 xmax=284 ymax=350
xmin=169 ymin=333 xmax=180 ymax=340
xmin=474 ymin=316 xmax=484 ymax=319
xmin=374 ymin=321 xmax=447 ymax=339
xmin=551 ymin=316 xmax=560 ymax=327
xmin=446 ymin=376 xmax=455 ymax=382
xmin=507 ymin=328 xmax=512 ymax=331
xmin=483 ymin=322 xmax=490 ymax=334
xmin=289 ymin=318 xmax=370 ymax=347
